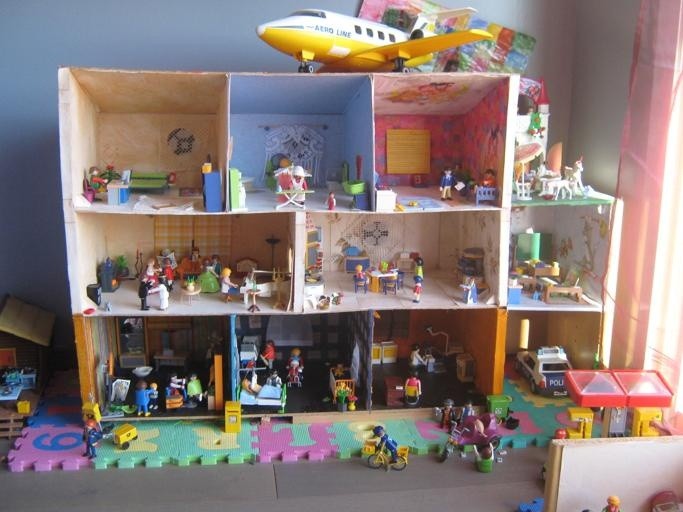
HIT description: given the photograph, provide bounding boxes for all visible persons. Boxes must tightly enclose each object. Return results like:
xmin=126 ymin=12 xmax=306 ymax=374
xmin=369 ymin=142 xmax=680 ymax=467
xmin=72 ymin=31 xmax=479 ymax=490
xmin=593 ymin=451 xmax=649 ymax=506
xmin=601 ymin=496 xmax=624 ymax=512
